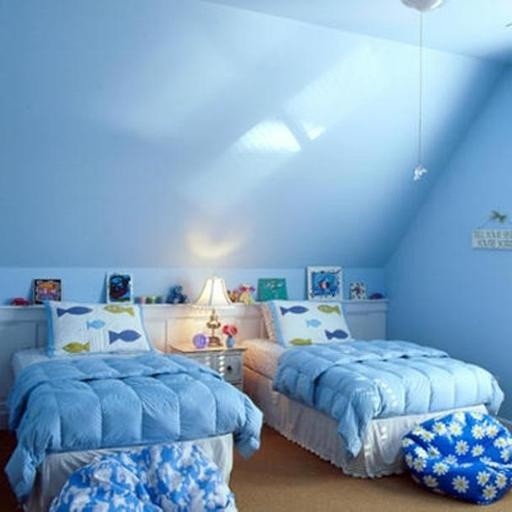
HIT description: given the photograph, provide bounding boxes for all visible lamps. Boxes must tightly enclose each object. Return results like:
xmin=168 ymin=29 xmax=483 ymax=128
xmin=190 ymin=276 xmax=236 ymax=346
xmin=402 ymin=0 xmax=446 ymax=13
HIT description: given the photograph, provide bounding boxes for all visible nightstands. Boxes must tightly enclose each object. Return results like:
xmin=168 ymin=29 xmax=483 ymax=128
xmin=170 ymin=343 xmax=248 ymax=391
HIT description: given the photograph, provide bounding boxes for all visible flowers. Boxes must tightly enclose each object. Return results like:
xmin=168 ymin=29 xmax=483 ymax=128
xmin=222 ymin=325 xmax=237 ymax=336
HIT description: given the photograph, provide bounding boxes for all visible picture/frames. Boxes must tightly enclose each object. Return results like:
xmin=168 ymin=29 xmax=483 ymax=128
xmin=306 ymin=265 xmax=344 ymax=302
xmin=32 ymin=277 xmax=63 ymax=305
xmin=105 ymin=272 xmax=134 ymax=303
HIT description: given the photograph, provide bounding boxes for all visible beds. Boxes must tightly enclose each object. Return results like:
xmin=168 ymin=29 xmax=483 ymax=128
xmin=11 ymin=347 xmax=245 ymax=511
xmin=238 ymin=339 xmax=503 ymax=480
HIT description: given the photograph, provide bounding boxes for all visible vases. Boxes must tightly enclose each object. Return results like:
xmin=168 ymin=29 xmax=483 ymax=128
xmin=224 ymin=335 xmax=234 ymax=347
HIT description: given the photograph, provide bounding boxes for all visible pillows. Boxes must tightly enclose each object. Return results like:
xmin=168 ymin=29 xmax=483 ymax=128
xmin=45 ymin=299 xmax=153 ymax=358
xmin=259 ymin=299 xmax=352 ymax=348
